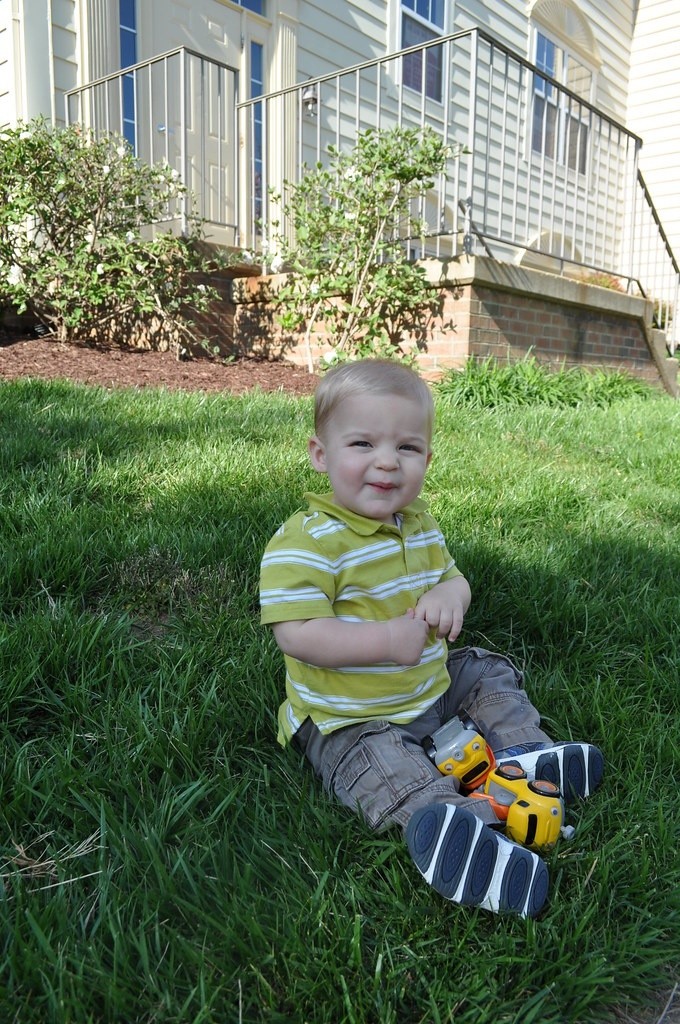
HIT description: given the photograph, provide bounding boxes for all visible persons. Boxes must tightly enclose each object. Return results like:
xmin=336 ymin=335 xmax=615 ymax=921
xmin=259 ymin=359 xmax=605 ymax=921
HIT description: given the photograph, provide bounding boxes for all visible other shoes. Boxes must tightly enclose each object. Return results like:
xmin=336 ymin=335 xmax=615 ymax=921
xmin=408 ymin=804 xmax=552 ymax=919
xmin=492 ymin=741 xmax=605 ymax=801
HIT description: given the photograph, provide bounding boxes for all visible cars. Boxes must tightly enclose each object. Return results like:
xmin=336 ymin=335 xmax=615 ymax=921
xmin=464 ymin=761 xmax=576 ymax=851
xmin=422 ymin=709 xmax=495 ymax=791
